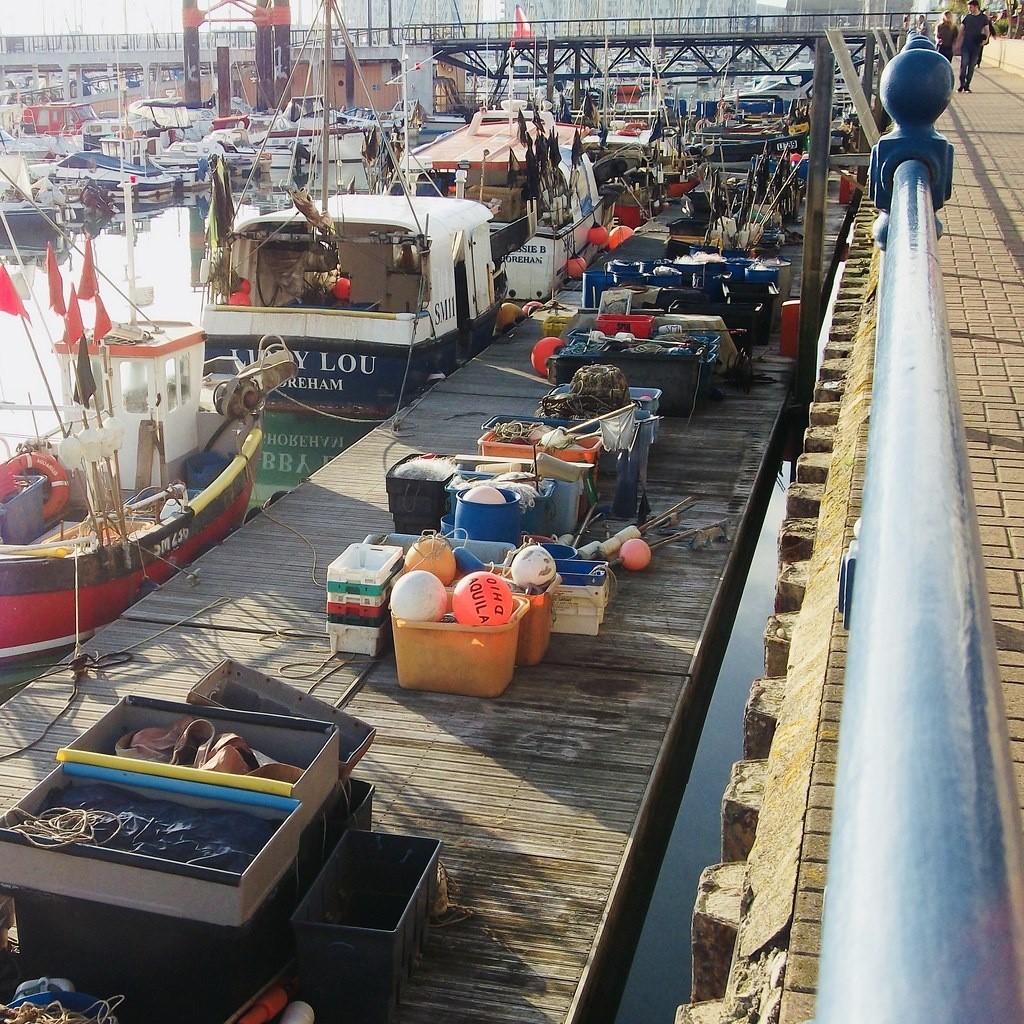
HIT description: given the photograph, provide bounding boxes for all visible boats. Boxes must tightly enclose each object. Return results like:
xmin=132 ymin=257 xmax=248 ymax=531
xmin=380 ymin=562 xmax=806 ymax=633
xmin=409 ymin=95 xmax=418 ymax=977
xmin=0 ymin=0 xmax=820 ymax=678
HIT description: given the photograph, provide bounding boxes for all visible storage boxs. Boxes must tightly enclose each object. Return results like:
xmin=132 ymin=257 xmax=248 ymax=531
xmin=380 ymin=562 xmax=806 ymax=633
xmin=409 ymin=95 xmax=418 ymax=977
xmin=362 ymin=533 xmax=516 ymax=567
xmin=110 ymin=486 xmax=204 ymax=522
xmin=41 ymin=520 xmax=155 ymax=545
xmin=288 ymin=827 xmax=445 ymax=1024
xmin=0 ymin=695 xmax=338 ymax=1024
xmin=327 ymin=584 xmax=392 ymax=607
xmin=481 ymin=414 xmax=602 ymax=437
xmin=327 ymin=558 xmax=404 ymax=597
xmin=449 ymin=565 xmax=562 ymax=665
xmin=454 ymin=463 xmax=584 ymax=538
xmin=0 ymin=474 xmax=48 ymax=546
xmin=477 ymin=431 xmax=602 ymax=518
xmin=326 ymin=616 xmax=391 ymax=657
xmin=385 ymin=454 xmax=458 ymax=535
xmin=554 ymin=559 xmax=609 ymax=586
xmin=544 ymin=155 xmax=808 ymax=442
xmin=298 ymin=779 xmax=376 ymax=899
xmin=326 ymin=542 xmax=403 ymax=585
xmin=327 ymin=598 xmax=391 ymax=617
xmin=387 ymin=591 xmax=531 ymax=699
xmin=550 ymin=575 xmax=608 ymax=636
xmin=327 ymin=611 xmax=388 ymax=628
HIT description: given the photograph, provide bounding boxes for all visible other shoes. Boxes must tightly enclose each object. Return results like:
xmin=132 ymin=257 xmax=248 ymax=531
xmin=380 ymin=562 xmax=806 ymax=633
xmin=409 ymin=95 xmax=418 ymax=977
xmin=958 ymin=85 xmax=964 ymax=92
xmin=964 ymin=86 xmax=972 ymax=93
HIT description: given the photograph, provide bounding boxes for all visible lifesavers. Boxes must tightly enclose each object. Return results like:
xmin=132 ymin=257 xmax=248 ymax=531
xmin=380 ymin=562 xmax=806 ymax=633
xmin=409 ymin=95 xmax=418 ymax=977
xmin=7 ymin=453 xmax=70 ymax=520
xmin=694 ymin=120 xmax=711 ymax=134
xmin=617 ymin=129 xmax=640 ymax=136
xmin=625 ymin=122 xmax=648 ymax=129
xmin=239 ymin=986 xmax=316 ymax=1024
xmin=122 ymin=126 xmax=134 ymax=140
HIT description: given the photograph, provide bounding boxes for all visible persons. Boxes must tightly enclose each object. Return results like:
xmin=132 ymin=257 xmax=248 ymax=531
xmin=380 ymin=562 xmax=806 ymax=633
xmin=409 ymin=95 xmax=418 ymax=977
xmin=918 ymin=0 xmax=996 ymax=94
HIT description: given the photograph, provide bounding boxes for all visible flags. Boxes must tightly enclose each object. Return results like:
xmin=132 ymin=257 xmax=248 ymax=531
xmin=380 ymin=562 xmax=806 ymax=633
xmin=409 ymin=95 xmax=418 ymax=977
xmin=0 ymin=234 xmax=113 ymax=406
